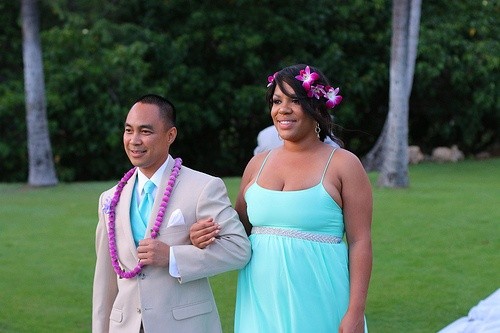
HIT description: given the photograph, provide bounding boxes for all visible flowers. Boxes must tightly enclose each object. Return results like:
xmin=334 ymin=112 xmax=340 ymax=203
xmin=267 ymin=65 xmax=342 ymax=108
xmin=102 ymin=196 xmax=113 ymax=214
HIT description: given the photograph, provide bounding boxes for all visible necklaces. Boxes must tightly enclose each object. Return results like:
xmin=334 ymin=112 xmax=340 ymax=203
xmin=108 ymin=158 xmax=182 ymax=278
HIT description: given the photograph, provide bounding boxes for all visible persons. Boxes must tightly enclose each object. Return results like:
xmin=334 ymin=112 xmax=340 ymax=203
xmin=190 ymin=65 xmax=373 ymax=333
xmin=91 ymin=94 xmax=253 ymax=333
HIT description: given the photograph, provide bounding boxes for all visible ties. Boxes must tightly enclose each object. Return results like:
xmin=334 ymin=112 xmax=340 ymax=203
xmin=139 ymin=181 xmax=156 ymax=229
